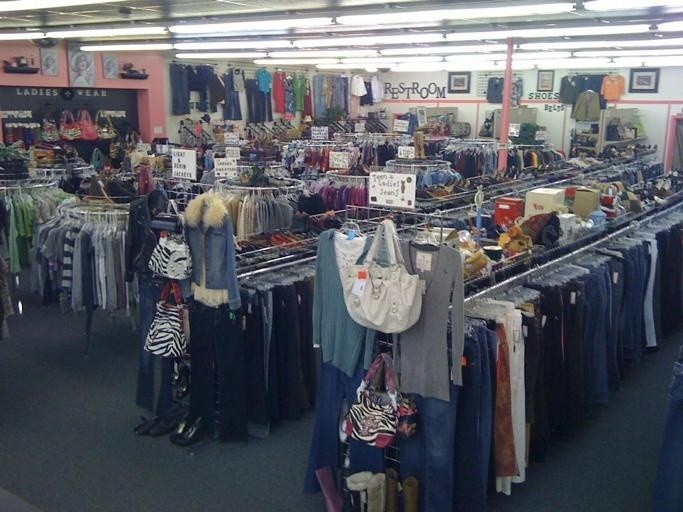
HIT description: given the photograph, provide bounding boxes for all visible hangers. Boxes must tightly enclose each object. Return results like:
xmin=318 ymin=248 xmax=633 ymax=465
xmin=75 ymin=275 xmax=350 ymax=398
xmin=1 ymin=108 xmax=682 ymax=359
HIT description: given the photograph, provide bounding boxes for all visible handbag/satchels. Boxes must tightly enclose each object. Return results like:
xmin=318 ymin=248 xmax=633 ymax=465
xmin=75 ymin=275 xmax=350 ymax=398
xmin=342 ymin=391 xmax=419 ymax=448
xmin=144 ymin=300 xmax=192 ymax=358
xmin=43 ymin=122 xmax=116 ymax=142
xmin=341 ymin=261 xmax=427 ymax=333
xmin=148 ymin=236 xmax=193 ymax=280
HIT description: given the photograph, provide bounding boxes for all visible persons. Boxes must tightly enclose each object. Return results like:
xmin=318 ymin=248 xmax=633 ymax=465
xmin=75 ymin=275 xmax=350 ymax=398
xmin=42 ymin=52 xmax=58 ymax=76
xmin=103 ymin=54 xmax=120 ymax=79
xmin=68 ymin=49 xmax=94 ymax=85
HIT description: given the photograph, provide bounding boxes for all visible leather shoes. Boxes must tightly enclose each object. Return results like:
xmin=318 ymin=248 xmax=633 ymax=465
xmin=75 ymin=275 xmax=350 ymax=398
xmin=134 ymin=407 xmax=206 ymax=446
xmin=171 ymin=360 xmax=190 ymax=399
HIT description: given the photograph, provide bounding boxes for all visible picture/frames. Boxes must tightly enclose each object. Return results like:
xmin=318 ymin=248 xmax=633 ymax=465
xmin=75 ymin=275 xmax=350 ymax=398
xmin=536 ymin=70 xmax=555 ymax=92
xmin=448 ymin=71 xmax=471 ymax=93
xmin=628 ymin=68 xmax=660 ymax=93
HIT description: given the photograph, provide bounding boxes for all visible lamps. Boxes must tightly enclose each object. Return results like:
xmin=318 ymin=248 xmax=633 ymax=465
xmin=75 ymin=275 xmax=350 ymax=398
xmin=0 ymin=0 xmax=683 ymax=66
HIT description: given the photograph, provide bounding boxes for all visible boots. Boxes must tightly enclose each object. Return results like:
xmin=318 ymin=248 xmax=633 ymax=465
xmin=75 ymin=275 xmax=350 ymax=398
xmin=314 ymin=465 xmax=419 ymax=512
